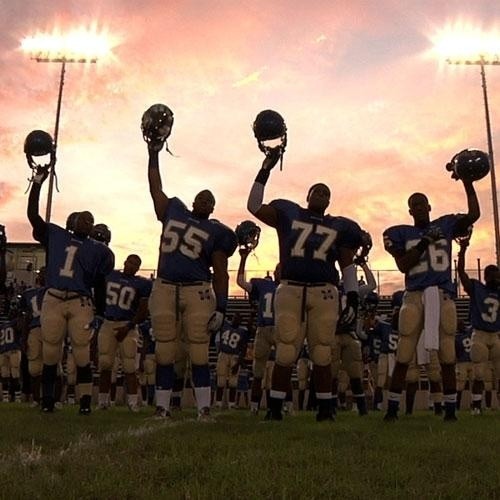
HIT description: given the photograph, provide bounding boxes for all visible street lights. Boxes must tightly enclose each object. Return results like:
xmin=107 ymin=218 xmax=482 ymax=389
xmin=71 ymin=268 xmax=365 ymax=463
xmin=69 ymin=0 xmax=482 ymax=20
xmin=28 ymin=28 xmax=98 ymax=224
xmin=444 ymin=36 xmax=500 ymax=273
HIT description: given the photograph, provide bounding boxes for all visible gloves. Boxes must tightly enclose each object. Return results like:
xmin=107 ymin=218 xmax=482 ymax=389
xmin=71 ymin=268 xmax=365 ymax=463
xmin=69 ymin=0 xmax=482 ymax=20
xmin=355 ymin=257 xmax=366 ymax=266
xmin=84 ymin=316 xmax=104 ymax=342
xmin=208 ymin=306 xmax=223 ymax=334
xmin=338 ymin=294 xmax=360 ymax=331
xmin=148 ymin=140 xmax=163 ymax=165
xmin=31 ymin=164 xmax=49 ymax=186
xmin=419 ymin=225 xmax=444 ymax=249
xmin=114 ymin=323 xmax=133 ymax=342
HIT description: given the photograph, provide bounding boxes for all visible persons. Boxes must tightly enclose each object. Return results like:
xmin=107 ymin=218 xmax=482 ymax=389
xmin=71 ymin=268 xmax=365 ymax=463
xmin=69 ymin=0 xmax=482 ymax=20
xmin=0 ymin=246 xmax=500 ymax=420
xmin=381 ymin=165 xmax=481 ymax=425
xmin=23 ymin=163 xmax=113 ymax=417
xmin=246 ymin=146 xmax=362 ymax=425
xmin=145 ymin=136 xmax=237 ymax=425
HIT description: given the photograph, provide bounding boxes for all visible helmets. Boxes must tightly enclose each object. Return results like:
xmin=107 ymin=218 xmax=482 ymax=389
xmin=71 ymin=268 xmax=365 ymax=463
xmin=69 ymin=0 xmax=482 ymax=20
xmin=66 ymin=211 xmax=94 ymax=230
xmin=35 ymin=267 xmax=45 ymax=278
xmin=364 ymin=291 xmax=405 ymax=307
xmin=447 ymin=149 xmax=490 ymax=181
xmin=90 ymin=222 xmax=110 ymax=244
xmin=140 ymin=103 xmax=174 ymax=147
xmin=23 ymin=129 xmax=58 ymax=170
xmin=235 ymin=220 xmax=261 ymax=252
xmin=253 ymin=110 xmax=287 ymax=154
xmin=453 ymin=225 xmax=473 ymax=244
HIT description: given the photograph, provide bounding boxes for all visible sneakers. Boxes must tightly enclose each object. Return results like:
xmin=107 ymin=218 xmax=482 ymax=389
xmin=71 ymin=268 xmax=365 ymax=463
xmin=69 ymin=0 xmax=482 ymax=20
xmin=0 ymin=386 xmax=499 ymax=427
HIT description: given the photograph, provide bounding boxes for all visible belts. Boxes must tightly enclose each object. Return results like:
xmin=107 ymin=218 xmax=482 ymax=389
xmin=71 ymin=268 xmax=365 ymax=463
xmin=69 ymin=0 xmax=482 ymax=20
xmin=380 ymin=351 xmax=394 ymax=355
xmin=45 ymin=291 xmax=85 ymax=303
xmin=222 ymin=350 xmax=239 ymax=356
xmin=258 ymin=324 xmax=275 ymax=327
xmin=406 ymin=284 xmax=444 ymax=292
xmin=368 ymin=359 xmax=378 ymax=362
xmin=286 ymin=280 xmax=329 ymax=287
xmin=472 ymin=326 xmax=500 ymax=334
xmin=105 ymin=315 xmax=130 ymax=321
xmin=457 ymin=359 xmax=472 ymax=363
xmin=160 ymin=279 xmax=204 ymax=288
xmin=28 ymin=325 xmax=41 ymax=330
xmin=335 ymin=331 xmax=350 ymax=335
xmin=0 ymin=348 xmax=22 ymax=354
